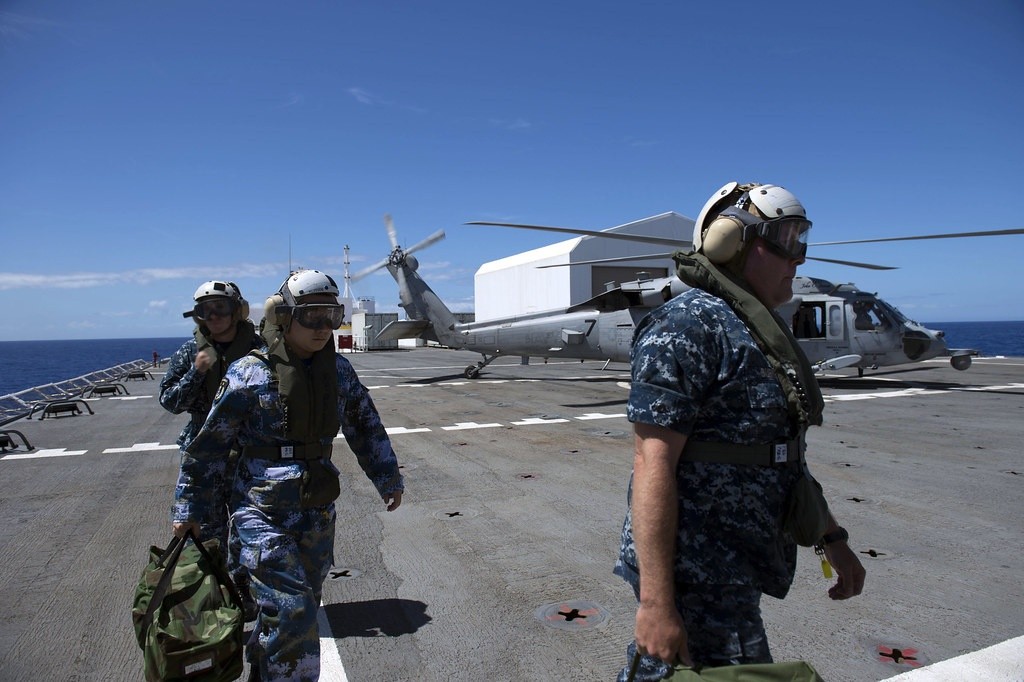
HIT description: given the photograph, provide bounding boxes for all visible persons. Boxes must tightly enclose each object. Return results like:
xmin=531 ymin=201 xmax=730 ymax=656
xmin=612 ymin=181 xmax=866 ymax=682
xmin=173 ymin=270 xmax=404 ymax=682
xmin=157 ymin=279 xmax=263 ymax=622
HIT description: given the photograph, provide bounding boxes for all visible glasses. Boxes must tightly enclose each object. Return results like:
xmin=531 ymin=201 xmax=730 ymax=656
xmin=758 ymin=215 xmax=813 ymax=260
xmin=183 ymin=298 xmax=237 ymax=321
xmin=290 ymin=304 xmax=345 ymax=329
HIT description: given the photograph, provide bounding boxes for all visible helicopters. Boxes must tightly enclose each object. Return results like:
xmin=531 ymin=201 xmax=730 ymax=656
xmin=349 ymin=212 xmax=1024 ymax=379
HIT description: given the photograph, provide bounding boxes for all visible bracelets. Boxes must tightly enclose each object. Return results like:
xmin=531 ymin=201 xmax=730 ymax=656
xmin=814 ymin=527 xmax=848 ymax=579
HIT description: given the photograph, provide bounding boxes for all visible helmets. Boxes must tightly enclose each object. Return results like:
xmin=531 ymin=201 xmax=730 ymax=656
xmin=193 ymin=280 xmax=244 ymax=301
xmin=692 ymin=181 xmax=807 ymax=278
xmin=277 ymin=269 xmax=340 ymax=297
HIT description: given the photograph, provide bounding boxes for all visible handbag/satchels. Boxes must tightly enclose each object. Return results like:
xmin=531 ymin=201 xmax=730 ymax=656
xmin=131 ymin=528 xmax=245 ymax=682
xmin=625 ymin=651 xmax=823 ymax=682
